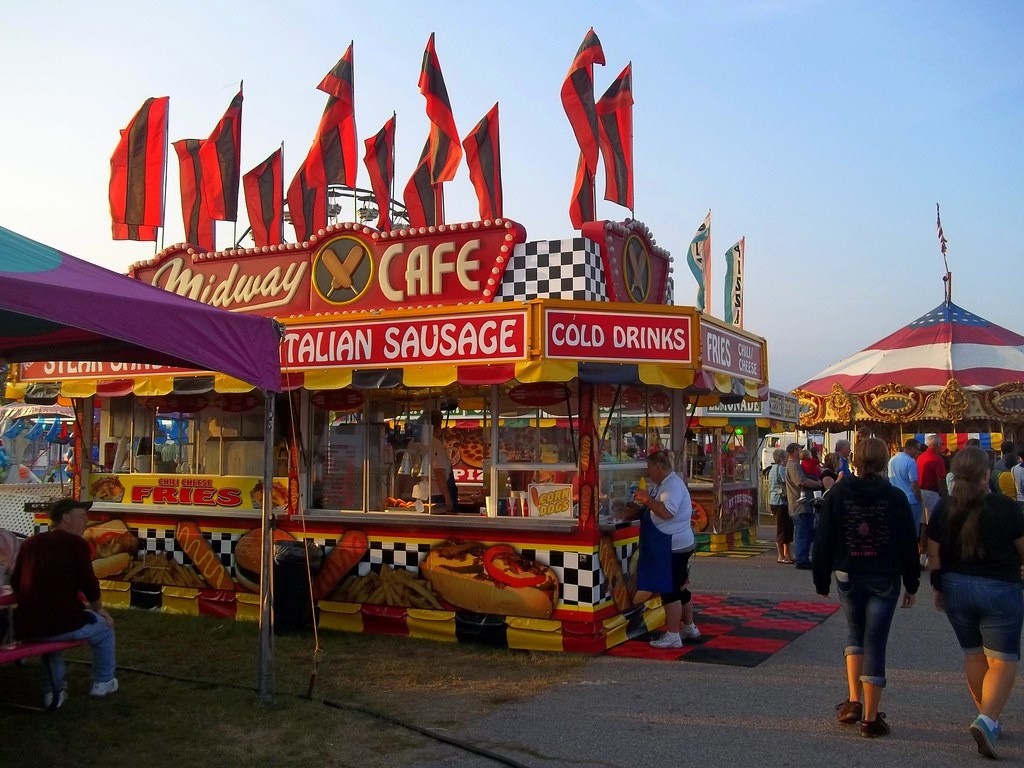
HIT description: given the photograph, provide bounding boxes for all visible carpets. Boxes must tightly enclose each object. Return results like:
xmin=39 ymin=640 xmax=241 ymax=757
xmin=604 ymin=589 xmax=841 ymax=667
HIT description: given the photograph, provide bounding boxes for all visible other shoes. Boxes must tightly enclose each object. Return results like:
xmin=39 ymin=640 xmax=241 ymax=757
xmin=796 ymin=563 xmax=812 ymax=570
xmin=969 ymin=718 xmax=1001 ymax=758
xmin=797 ymin=497 xmax=806 ymax=503
xmin=777 ymin=557 xmax=795 ymax=564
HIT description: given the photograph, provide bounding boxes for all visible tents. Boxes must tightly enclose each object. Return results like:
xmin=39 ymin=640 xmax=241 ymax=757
xmin=0 ymin=224 xmax=286 ymax=703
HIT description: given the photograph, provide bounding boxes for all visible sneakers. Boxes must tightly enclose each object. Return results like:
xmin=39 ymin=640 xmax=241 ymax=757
xmin=680 ymin=623 xmax=701 ymax=638
xmin=835 ymin=699 xmax=862 ymax=723
xmin=45 ymin=689 xmax=64 ymax=708
xmin=650 ymin=633 xmax=683 ymax=649
xmin=90 ymin=678 xmax=118 ymax=696
xmin=861 ymin=712 xmax=890 ymax=737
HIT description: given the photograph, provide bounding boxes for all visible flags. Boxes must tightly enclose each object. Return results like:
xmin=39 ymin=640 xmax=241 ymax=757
xmin=241 ymin=43 xmax=358 ymax=250
xmin=109 ymin=96 xmax=169 ymax=242
xmin=937 ymin=218 xmax=947 ymax=243
xmin=560 ymin=28 xmax=634 ymax=230
xmin=363 ymin=35 xmax=502 ymax=231
xmin=686 ymin=212 xmax=711 ymax=313
xmin=724 ymin=238 xmax=744 ymax=329
xmin=170 ymin=139 xmax=214 ymax=252
xmin=198 ymin=90 xmax=244 ymax=222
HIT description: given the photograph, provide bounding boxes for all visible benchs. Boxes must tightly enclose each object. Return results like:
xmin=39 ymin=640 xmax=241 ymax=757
xmin=0 ymin=640 xmax=90 ymax=714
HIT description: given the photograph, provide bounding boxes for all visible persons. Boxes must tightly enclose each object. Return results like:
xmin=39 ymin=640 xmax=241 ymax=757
xmin=686 ymin=428 xmax=706 ymax=476
xmin=398 ymin=410 xmax=459 ymax=512
xmin=927 ymin=446 xmax=1024 ymax=757
xmin=811 ymin=439 xmax=921 ymax=736
xmin=610 ymin=452 xmax=701 ymax=646
xmin=10 ymin=498 xmax=118 ymax=708
xmin=48 ymin=474 xmax=56 ymax=482
xmin=65 ymin=438 xmax=74 ymax=481
xmin=768 ymin=427 xmax=1024 ymax=570
xmin=627 ymin=434 xmax=645 ymax=457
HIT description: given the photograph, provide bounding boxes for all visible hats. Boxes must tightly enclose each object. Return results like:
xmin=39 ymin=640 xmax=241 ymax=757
xmin=787 ymin=443 xmax=805 ymax=452
xmin=50 ymin=498 xmax=93 ymax=519
